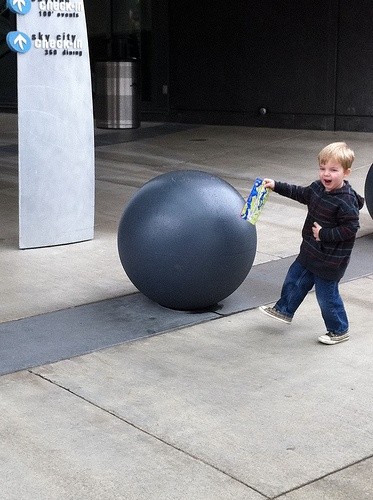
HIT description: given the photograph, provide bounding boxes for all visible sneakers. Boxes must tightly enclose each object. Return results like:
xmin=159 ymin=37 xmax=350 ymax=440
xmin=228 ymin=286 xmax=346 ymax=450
xmin=318 ymin=331 xmax=349 ymax=345
xmin=259 ymin=305 xmax=292 ymax=325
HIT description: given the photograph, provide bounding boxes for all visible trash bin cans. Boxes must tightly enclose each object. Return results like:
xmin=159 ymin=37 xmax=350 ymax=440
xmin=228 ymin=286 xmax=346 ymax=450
xmin=96 ymin=57 xmax=141 ymax=129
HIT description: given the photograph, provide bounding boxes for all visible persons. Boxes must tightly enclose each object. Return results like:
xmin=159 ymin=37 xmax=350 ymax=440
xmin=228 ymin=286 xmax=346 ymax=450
xmin=257 ymin=141 xmax=364 ymax=345
